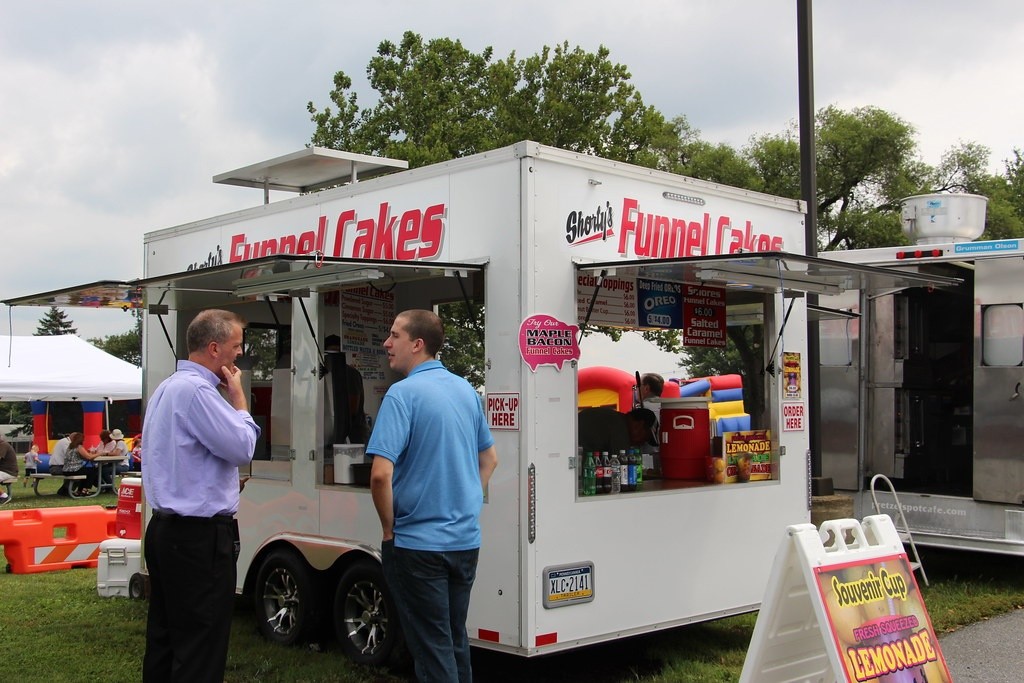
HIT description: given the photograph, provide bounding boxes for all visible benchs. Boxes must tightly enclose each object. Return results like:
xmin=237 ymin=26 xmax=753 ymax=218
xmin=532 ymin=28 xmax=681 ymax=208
xmin=109 ymin=470 xmax=142 ymax=495
xmin=0 ymin=478 xmax=17 ymax=498
xmin=29 ymin=473 xmax=87 ymax=498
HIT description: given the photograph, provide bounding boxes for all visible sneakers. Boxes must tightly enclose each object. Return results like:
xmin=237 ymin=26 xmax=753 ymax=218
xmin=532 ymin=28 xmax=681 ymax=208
xmin=0 ymin=495 xmax=12 ymax=505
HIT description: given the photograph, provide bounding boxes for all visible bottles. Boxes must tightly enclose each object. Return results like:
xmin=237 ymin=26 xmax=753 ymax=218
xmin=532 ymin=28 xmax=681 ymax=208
xmin=627 ymin=450 xmax=637 ymax=491
xmin=582 ymin=452 xmax=596 ymax=495
xmin=593 ymin=452 xmax=604 ymax=494
xmin=634 ymin=449 xmax=643 ymax=485
xmin=609 ymin=455 xmax=620 ymax=493
xmin=618 ymin=450 xmax=628 ymax=492
xmin=601 ymin=452 xmax=613 ymax=493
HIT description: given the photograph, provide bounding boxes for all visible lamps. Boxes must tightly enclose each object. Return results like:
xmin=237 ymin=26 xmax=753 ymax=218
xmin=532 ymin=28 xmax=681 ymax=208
xmin=232 ymin=269 xmax=384 ymax=299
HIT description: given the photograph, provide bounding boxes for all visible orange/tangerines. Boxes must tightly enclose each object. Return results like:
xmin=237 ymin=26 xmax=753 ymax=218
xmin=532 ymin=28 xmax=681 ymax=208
xmin=714 ymin=459 xmax=727 ymax=483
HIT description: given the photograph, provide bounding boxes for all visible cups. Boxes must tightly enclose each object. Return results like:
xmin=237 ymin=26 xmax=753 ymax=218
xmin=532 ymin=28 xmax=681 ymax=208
xmin=735 ymin=451 xmax=753 ymax=483
xmin=704 ymin=455 xmax=725 ymax=485
xmin=89 ymin=447 xmax=95 ymax=455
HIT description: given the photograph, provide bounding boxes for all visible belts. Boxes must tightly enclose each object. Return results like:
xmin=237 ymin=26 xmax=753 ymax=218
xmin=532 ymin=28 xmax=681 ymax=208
xmin=152 ymin=508 xmax=233 ymax=519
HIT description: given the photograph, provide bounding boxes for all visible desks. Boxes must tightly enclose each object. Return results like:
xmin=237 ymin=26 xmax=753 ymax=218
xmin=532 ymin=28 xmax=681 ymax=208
xmin=86 ymin=455 xmax=130 ymax=497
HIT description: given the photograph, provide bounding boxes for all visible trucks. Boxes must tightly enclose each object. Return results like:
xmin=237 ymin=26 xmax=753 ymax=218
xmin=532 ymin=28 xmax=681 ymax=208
xmin=0 ymin=139 xmax=965 ymax=670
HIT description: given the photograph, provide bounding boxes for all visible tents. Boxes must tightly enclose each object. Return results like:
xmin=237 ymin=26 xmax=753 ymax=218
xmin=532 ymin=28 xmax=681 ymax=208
xmin=0 ymin=333 xmax=143 ymax=430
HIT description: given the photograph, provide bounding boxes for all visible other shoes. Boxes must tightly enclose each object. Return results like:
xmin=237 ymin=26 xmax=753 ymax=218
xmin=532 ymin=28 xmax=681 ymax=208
xmin=71 ymin=492 xmax=80 ymax=496
xmin=104 ymin=487 xmax=116 ymax=494
xmin=58 ymin=489 xmax=70 ymax=497
xmin=82 ymin=490 xmax=92 ymax=496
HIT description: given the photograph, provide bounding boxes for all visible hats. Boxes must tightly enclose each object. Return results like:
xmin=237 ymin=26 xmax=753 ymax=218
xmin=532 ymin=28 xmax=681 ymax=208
xmin=109 ymin=429 xmax=124 ymax=440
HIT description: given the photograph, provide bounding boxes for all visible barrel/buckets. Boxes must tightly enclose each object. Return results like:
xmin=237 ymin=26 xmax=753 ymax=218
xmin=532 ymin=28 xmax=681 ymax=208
xmin=648 ymin=396 xmax=715 ymax=480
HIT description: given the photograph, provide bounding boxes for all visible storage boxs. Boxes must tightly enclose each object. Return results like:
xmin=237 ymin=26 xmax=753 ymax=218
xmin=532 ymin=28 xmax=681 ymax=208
xmin=96 ymin=537 xmax=144 ymax=599
xmin=0 ymin=505 xmax=108 ymax=574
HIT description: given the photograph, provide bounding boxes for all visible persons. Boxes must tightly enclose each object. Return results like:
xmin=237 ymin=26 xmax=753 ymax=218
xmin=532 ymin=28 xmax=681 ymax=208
xmin=324 ymin=333 xmax=364 ymax=444
xmin=49 ymin=428 xmax=141 ymax=496
xmin=23 ymin=444 xmax=42 ymax=487
xmin=365 ymin=308 xmax=498 ymax=683
xmin=0 ymin=436 xmax=18 ymax=505
xmin=578 ymin=372 xmax=664 ymax=459
xmin=140 ymin=308 xmax=261 ymax=683
